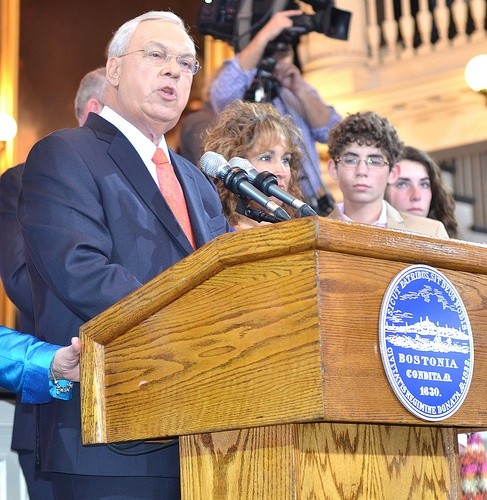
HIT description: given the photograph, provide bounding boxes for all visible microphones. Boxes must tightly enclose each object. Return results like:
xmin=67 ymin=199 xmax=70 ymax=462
xmin=228 ymin=157 xmax=318 ymax=216
xmin=200 ymin=151 xmax=291 ymax=222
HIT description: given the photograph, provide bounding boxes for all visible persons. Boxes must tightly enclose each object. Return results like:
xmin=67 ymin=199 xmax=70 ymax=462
xmin=200 ymin=101 xmax=306 ymax=231
xmin=17 ymin=12 xmax=235 ymax=500
xmin=0 ymin=326 xmax=83 ymax=404
xmin=1 ymin=67 xmax=118 ymax=500
xmin=210 ymin=10 xmax=342 ymax=216
xmin=385 ymin=146 xmax=458 ymax=240
xmin=326 ymin=111 xmax=451 ymax=239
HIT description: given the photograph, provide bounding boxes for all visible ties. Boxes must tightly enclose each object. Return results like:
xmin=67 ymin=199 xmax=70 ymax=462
xmin=151 ymin=148 xmax=197 ymax=252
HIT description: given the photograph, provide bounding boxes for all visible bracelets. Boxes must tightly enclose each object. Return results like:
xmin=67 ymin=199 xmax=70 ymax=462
xmin=50 ymin=363 xmax=74 ymax=395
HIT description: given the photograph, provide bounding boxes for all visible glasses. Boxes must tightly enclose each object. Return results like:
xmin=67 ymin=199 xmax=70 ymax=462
xmin=118 ymin=44 xmax=202 ymax=76
xmin=335 ymin=154 xmax=389 ymax=168
